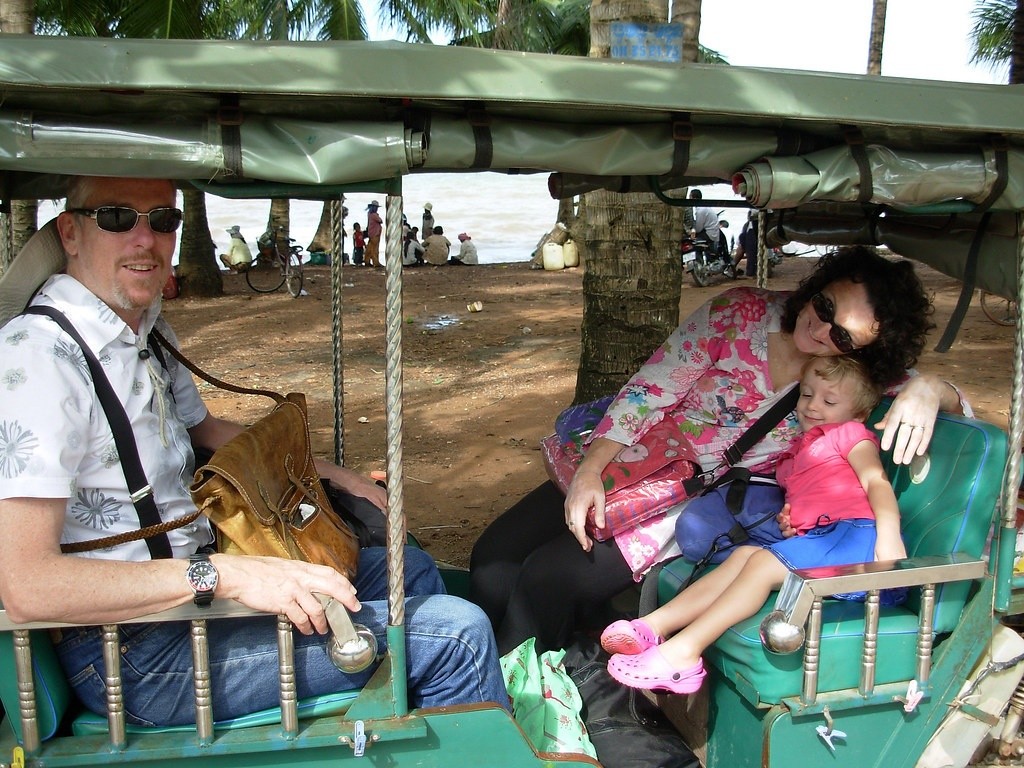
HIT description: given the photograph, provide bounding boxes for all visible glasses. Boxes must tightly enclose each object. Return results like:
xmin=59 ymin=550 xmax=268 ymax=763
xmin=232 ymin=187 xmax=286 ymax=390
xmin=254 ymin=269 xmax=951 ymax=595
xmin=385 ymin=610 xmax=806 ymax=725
xmin=812 ymin=292 xmax=863 ymax=354
xmin=71 ymin=206 xmax=185 ymax=234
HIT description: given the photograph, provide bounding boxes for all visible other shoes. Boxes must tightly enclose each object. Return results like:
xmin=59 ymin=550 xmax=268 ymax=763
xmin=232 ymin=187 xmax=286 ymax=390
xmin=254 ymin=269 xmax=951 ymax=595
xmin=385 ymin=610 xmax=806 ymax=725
xmin=365 ymin=263 xmax=373 ymax=267
xmin=374 ymin=262 xmax=384 ymax=267
xmin=724 ymin=270 xmax=733 ymax=277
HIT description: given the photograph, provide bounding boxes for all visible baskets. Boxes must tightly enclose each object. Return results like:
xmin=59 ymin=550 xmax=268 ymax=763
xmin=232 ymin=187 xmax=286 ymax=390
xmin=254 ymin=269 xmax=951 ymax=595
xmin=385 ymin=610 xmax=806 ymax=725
xmin=257 ymin=238 xmax=275 ymax=257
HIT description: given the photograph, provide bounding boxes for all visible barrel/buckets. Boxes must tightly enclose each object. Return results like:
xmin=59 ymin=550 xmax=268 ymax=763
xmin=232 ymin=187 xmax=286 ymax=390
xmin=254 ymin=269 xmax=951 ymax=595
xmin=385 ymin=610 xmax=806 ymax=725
xmin=564 ymin=240 xmax=578 ymax=267
xmin=542 ymin=242 xmax=565 ymax=270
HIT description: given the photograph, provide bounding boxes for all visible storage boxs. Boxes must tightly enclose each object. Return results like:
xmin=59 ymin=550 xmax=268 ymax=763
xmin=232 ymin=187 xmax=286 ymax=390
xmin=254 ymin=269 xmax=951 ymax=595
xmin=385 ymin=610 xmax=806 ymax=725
xmin=326 ymin=253 xmax=349 ymax=265
xmin=311 ymin=252 xmax=326 ymax=264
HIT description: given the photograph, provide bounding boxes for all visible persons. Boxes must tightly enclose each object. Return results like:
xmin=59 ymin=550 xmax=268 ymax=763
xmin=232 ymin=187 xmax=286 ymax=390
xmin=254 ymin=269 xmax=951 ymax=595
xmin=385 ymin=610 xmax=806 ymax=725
xmin=688 ymin=189 xmax=783 ymax=277
xmin=352 ymin=200 xmax=478 ymax=266
xmin=0 ymin=176 xmax=512 ymax=729
xmin=601 ymin=353 xmax=907 ymax=693
xmin=472 ymin=242 xmax=977 ymax=658
xmin=220 ymin=226 xmax=252 ymax=275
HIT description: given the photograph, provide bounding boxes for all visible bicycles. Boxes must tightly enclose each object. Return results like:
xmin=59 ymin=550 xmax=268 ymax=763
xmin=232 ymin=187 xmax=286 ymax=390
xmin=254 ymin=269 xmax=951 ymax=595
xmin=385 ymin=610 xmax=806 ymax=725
xmin=245 ymin=217 xmax=303 ymax=298
xmin=979 ymin=291 xmax=1016 ymax=326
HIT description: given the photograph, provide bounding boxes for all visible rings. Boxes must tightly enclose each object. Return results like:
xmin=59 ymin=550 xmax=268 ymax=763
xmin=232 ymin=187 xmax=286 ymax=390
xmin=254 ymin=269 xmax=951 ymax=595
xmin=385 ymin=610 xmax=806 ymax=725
xmin=913 ymin=426 xmax=924 ymax=432
xmin=901 ymin=421 xmax=914 ymax=428
xmin=568 ymin=521 xmax=575 ymax=526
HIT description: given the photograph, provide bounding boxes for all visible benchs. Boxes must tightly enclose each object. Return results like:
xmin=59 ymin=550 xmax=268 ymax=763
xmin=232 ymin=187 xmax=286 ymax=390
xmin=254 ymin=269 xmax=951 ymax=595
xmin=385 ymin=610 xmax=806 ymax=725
xmin=0 ymin=532 xmax=423 ymax=747
xmin=659 ymin=398 xmax=1010 ymax=709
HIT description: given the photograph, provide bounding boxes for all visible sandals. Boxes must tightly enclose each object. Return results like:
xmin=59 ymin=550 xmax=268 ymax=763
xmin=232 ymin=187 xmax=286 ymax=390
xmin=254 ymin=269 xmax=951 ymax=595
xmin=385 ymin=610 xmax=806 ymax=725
xmin=601 ymin=620 xmax=665 ymax=655
xmin=607 ymin=645 xmax=707 ymax=694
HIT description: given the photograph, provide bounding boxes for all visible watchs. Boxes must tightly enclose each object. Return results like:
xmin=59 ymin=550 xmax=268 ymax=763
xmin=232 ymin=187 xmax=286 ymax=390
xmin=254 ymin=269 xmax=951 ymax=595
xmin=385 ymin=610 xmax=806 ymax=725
xmin=187 ymin=553 xmax=219 ymax=608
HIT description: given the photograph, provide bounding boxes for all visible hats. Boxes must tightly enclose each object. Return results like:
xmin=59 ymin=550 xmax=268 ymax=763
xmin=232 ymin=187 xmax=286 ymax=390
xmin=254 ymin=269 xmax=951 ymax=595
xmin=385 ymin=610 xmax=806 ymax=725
xmin=226 ymin=226 xmax=244 ymax=238
xmin=402 ymin=214 xmax=407 ymax=220
xmin=368 ymin=200 xmax=381 ymax=207
xmin=0 ymin=217 xmax=67 ymax=329
xmin=425 ymin=203 xmax=432 ymax=211
xmin=458 ymin=233 xmax=471 ymax=240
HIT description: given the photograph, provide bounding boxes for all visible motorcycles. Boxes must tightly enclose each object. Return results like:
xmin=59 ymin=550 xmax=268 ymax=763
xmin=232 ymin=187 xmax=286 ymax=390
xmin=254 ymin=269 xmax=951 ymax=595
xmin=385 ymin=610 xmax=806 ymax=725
xmin=681 ymin=209 xmax=737 ymax=287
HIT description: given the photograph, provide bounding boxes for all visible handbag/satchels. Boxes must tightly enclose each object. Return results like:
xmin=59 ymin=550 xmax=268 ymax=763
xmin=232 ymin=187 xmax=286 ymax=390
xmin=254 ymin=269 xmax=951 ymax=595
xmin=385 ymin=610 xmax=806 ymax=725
xmin=189 ymin=392 xmax=359 ymax=585
xmin=674 ymin=468 xmax=786 ymax=565
xmin=499 ymin=637 xmax=599 ymax=762
xmin=541 ymin=394 xmax=705 ymax=542
